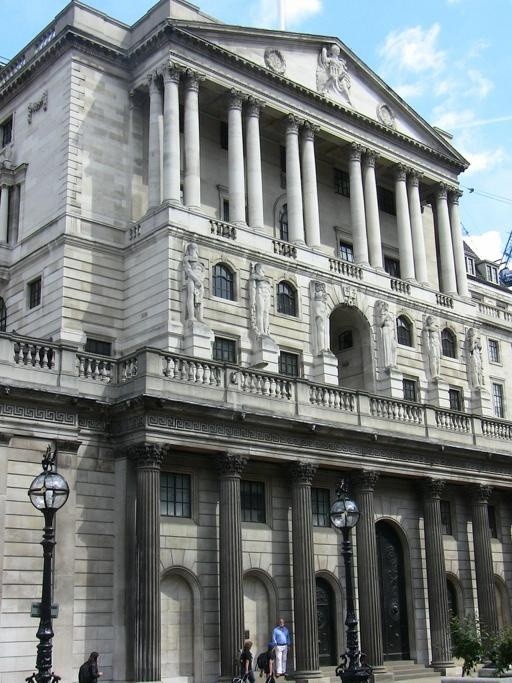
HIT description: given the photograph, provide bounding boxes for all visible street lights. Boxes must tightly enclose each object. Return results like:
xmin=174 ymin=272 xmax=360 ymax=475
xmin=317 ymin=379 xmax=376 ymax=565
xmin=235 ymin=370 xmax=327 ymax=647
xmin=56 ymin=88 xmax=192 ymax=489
xmin=328 ymin=474 xmax=372 ymax=681
xmin=18 ymin=441 xmax=76 ymax=683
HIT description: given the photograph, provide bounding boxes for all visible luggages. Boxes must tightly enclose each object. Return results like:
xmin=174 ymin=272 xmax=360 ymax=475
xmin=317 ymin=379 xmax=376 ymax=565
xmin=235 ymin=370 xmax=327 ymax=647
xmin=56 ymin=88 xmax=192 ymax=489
xmin=232 ymin=672 xmax=245 ymax=683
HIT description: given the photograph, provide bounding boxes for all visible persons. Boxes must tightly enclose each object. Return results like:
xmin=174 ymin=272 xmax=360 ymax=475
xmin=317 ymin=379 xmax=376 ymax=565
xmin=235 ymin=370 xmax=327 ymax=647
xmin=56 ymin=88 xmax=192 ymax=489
xmin=79 ymin=652 xmax=103 ymax=682
xmin=422 ymin=316 xmax=442 ymax=380
xmin=264 ymin=641 xmax=276 ymax=683
xmin=374 ymin=300 xmax=400 ymax=372
xmin=309 ymin=279 xmax=335 ymax=357
xmin=270 ymin=616 xmax=292 ymax=677
xmin=322 ymin=42 xmax=352 ymax=105
xmin=465 ymin=326 xmax=486 ymax=388
xmin=239 ymin=638 xmax=255 ymax=682
xmin=248 ymin=260 xmax=271 ymax=337
xmin=183 ymin=243 xmax=204 ymax=322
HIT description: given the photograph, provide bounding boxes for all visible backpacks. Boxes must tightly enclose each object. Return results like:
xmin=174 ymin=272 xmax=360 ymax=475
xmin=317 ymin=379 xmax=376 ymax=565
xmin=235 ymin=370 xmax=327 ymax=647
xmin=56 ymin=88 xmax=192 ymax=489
xmin=257 ymin=652 xmax=267 ymax=669
xmin=79 ymin=661 xmax=92 ymax=682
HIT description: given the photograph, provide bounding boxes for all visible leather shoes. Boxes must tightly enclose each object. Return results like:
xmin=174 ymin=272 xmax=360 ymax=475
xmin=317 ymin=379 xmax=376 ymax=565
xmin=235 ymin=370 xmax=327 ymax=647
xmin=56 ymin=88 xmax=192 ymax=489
xmin=276 ymin=673 xmax=281 ymax=676
xmin=283 ymin=672 xmax=289 ymax=676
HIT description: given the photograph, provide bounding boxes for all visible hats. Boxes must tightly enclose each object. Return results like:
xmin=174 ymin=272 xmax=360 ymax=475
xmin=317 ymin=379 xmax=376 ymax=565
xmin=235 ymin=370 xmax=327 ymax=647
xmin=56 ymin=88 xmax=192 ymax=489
xmin=268 ymin=642 xmax=275 ymax=649
